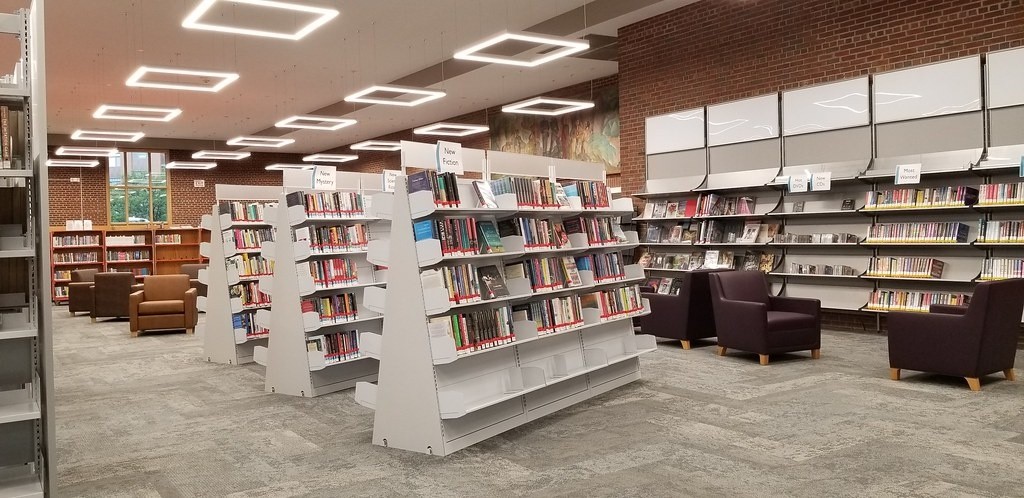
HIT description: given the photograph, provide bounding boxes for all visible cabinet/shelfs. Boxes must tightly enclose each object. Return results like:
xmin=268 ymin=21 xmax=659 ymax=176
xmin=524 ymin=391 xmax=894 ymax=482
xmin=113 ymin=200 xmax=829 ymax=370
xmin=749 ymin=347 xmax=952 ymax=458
xmin=353 ymin=141 xmax=657 ymax=457
xmin=765 ymin=73 xmax=881 ymax=333
xmin=630 ymin=105 xmax=709 ymax=296
xmin=969 ymin=45 xmax=1024 ymax=324
xmin=251 ymin=168 xmax=403 ymax=399
xmin=1 ymin=0 xmax=58 ymax=498
xmin=49 ymin=229 xmax=211 ymax=306
xmin=692 ymin=90 xmax=786 ymax=298
xmin=857 ymin=54 xmax=988 ymax=333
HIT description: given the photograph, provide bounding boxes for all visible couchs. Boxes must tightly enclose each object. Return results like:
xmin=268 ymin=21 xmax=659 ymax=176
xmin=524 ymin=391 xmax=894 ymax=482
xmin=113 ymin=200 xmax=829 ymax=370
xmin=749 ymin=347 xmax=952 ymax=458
xmin=129 ymin=274 xmax=198 ymax=338
xmin=885 ymin=277 xmax=1024 ymax=391
xmin=640 ymin=268 xmax=739 ymax=350
xmin=709 ymin=269 xmax=821 ymax=366
xmin=68 ymin=268 xmax=99 ymax=317
xmin=179 ymin=264 xmax=210 ymax=313
xmin=632 ymin=285 xmax=655 ymax=328
xmin=89 ymin=272 xmax=145 ymax=324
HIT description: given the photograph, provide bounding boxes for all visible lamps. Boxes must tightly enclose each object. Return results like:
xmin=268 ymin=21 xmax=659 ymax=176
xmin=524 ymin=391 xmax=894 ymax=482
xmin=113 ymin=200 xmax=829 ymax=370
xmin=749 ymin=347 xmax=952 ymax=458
xmin=46 ymin=0 xmax=596 ymax=171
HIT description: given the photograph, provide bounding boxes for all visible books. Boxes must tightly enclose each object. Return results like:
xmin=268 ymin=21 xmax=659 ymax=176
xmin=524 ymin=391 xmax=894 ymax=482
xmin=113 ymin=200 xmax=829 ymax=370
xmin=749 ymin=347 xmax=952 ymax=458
xmin=637 ymin=194 xmax=778 ymax=296
xmin=219 ymin=201 xmax=278 ymax=338
xmin=409 ymin=170 xmax=644 ymax=360
xmin=0 ymin=105 xmax=34 ymax=307
xmin=52 ymin=235 xmax=152 ymax=297
xmin=775 ymin=198 xmax=858 ymax=277
xmin=866 ymin=183 xmax=1024 ymax=322
xmin=155 ymin=234 xmax=181 ymax=244
xmin=286 ymin=190 xmax=370 ymax=366
